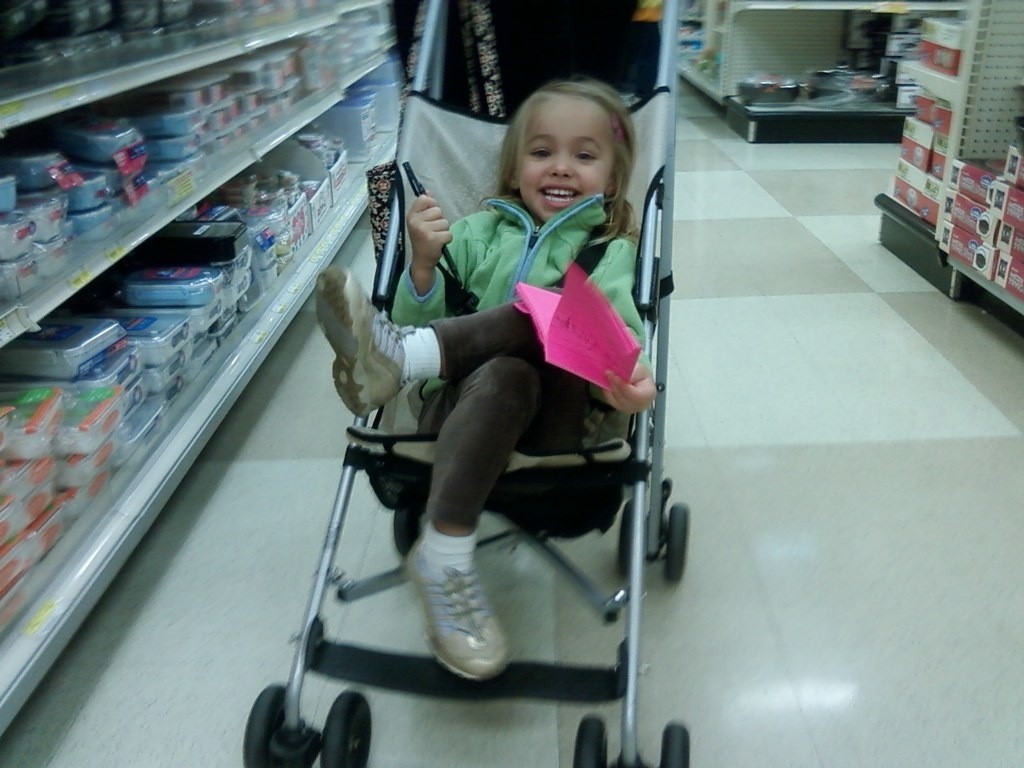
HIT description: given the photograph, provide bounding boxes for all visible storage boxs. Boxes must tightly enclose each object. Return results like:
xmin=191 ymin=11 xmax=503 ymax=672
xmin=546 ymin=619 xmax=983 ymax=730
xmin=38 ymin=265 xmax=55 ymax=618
xmin=851 ymin=9 xmax=1024 ymax=302
xmin=0 ymin=0 xmax=400 ymax=618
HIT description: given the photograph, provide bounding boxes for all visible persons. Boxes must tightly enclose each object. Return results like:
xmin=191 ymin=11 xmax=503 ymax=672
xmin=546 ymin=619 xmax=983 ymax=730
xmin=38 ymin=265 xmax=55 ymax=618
xmin=313 ymin=78 xmax=656 ymax=687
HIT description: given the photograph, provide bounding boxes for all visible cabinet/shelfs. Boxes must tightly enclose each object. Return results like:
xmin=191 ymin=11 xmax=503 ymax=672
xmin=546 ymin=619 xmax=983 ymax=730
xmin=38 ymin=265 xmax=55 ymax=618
xmin=0 ymin=0 xmax=405 ymax=737
xmin=874 ymin=0 xmax=1024 ymax=314
xmin=676 ymin=0 xmax=961 ymax=142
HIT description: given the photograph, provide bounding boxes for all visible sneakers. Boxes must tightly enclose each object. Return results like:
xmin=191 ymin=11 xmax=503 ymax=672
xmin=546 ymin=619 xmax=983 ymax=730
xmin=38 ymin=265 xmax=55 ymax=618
xmin=405 ymin=537 xmax=512 ymax=681
xmin=316 ymin=267 xmax=416 ymax=418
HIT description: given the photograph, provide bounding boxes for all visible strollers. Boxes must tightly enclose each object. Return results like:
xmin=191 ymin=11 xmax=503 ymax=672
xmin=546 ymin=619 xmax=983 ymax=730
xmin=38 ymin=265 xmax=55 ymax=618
xmin=236 ymin=1 xmax=692 ymax=768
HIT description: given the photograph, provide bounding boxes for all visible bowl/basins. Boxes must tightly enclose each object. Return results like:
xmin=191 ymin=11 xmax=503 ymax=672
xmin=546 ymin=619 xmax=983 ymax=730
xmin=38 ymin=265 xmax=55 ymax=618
xmin=0 ymin=1 xmax=394 ymax=305
xmin=737 ymin=82 xmax=798 ymax=106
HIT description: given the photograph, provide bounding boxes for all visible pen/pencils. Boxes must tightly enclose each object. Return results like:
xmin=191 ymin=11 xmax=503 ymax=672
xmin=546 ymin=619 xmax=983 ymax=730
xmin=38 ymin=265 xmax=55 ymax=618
xmin=403 ymin=161 xmax=463 ymax=285
